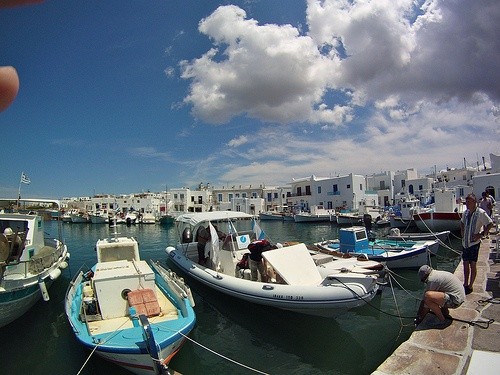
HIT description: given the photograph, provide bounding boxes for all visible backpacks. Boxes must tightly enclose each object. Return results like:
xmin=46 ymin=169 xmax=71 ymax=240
xmin=247 ymin=239 xmax=273 ymax=252
xmin=238 ymin=253 xmax=250 ymax=269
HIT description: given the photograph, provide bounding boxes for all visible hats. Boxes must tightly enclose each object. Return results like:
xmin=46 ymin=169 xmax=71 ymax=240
xmin=418 ymin=265 xmax=432 ymax=281
xmin=275 ymin=243 xmax=283 ymax=249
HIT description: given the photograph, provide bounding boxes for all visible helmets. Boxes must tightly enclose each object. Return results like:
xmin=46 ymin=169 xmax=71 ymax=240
xmin=4 ymin=228 xmax=13 ymax=235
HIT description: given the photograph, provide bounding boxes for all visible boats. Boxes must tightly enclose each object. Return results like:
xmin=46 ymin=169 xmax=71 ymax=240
xmin=61 ymin=209 xmax=156 ymax=224
xmin=0 ymin=176 xmax=71 ymax=328
xmin=165 ymin=177 xmax=466 ymax=320
xmin=65 ymin=197 xmax=195 ymax=375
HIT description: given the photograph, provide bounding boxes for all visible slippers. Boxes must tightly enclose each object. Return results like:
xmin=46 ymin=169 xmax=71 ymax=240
xmin=427 ymin=316 xmax=445 ymax=325
xmin=440 ymin=307 xmax=449 ymax=316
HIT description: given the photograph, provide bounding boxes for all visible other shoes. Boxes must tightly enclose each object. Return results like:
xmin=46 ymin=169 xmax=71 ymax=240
xmin=463 ymin=284 xmax=473 ymax=293
xmin=486 ymin=235 xmax=489 ymax=239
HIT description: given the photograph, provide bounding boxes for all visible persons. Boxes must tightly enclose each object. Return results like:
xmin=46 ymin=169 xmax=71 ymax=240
xmin=415 ymin=265 xmax=465 ymax=328
xmin=479 ymin=191 xmax=496 ymax=236
xmin=459 ymin=193 xmax=493 ymax=294
xmin=196 ymin=224 xmax=214 ymax=268
xmin=247 ymin=238 xmax=276 ymax=283
